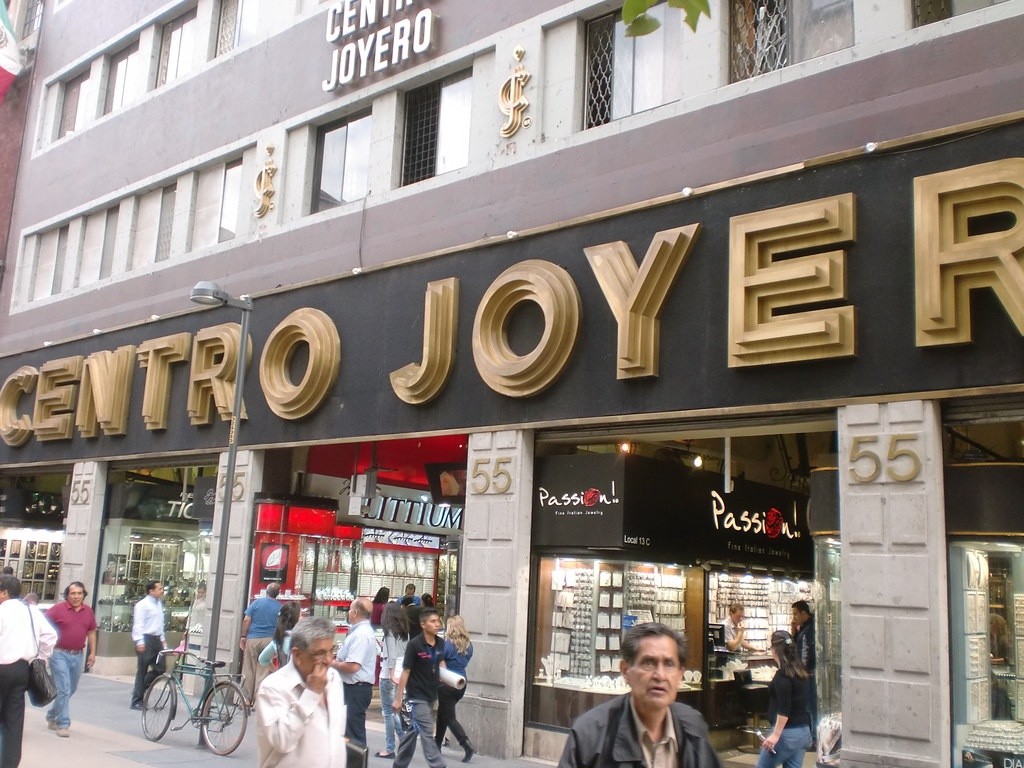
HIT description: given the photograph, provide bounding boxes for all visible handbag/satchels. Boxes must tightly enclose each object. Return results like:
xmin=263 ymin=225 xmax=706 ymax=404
xmin=27 ymin=660 xmax=58 ymax=708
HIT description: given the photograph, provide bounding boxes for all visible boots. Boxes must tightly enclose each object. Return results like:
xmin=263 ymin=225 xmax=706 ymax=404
xmin=461 ymin=739 xmax=476 ymax=763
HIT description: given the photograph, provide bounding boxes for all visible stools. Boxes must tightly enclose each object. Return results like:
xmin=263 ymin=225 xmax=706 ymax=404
xmin=733 ymin=669 xmax=771 ymax=755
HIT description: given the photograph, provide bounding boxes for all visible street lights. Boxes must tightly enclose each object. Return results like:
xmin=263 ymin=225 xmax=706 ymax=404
xmin=190 ymin=279 xmax=252 ymax=743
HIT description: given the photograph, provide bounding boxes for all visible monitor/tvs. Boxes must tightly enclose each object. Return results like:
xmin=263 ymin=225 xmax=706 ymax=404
xmin=708 ymin=623 xmax=725 ymax=654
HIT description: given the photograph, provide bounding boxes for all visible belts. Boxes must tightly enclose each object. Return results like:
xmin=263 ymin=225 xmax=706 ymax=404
xmin=54 ymin=647 xmax=83 ymax=654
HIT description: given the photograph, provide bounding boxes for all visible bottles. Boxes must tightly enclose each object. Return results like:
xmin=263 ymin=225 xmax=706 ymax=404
xmin=757 ymin=731 xmax=777 ymax=756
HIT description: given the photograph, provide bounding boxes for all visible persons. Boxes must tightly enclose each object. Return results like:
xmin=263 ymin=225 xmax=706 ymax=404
xmin=330 ymin=598 xmax=377 ymax=746
xmin=757 ymin=631 xmax=811 ymax=768
xmin=254 ymin=617 xmax=347 ymax=768
xmin=370 ymin=583 xmax=435 ymax=639
xmin=791 ymin=601 xmax=818 ymax=751
xmin=392 ymin=606 xmax=448 ymax=768
xmin=131 ymin=580 xmax=167 ymax=711
xmin=376 ymin=601 xmax=410 ymax=760
xmin=723 ymin=603 xmax=756 ymax=651
xmin=434 ymin=615 xmax=475 ymax=762
xmin=557 ymin=623 xmax=723 ymax=768
xmin=44 ymin=581 xmax=97 ymax=738
xmin=24 ymin=594 xmax=39 ymax=607
xmin=184 ymin=580 xmax=207 ymax=635
xmin=240 ymin=582 xmax=283 ymax=712
xmin=258 ymin=601 xmax=303 ymax=671
xmin=0 ymin=575 xmax=58 ymax=768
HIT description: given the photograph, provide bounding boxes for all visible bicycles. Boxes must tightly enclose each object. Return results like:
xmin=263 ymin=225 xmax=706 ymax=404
xmin=142 ymin=648 xmax=252 ymax=757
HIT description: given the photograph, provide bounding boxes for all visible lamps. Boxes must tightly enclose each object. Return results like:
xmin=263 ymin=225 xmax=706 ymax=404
xmin=783 ymin=567 xmax=801 ymax=585
xmin=744 ymin=564 xmax=754 ymax=579
xmin=763 ymin=565 xmax=775 ymax=582
xmin=719 ymin=560 xmax=730 ymax=577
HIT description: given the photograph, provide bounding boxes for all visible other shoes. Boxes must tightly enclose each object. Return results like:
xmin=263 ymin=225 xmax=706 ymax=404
xmin=130 ymin=702 xmax=147 ymax=711
xmin=375 ymin=752 xmax=396 ymax=759
xmin=45 ymin=715 xmax=57 ymax=730
xmin=56 ymin=727 xmax=69 ymax=738
xmin=807 ymin=744 xmax=817 ymax=752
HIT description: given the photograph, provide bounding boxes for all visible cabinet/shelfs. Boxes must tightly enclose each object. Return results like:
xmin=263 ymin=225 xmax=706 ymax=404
xmin=93 ymin=528 xmax=194 ymax=658
xmin=315 ymin=599 xmax=353 ymax=634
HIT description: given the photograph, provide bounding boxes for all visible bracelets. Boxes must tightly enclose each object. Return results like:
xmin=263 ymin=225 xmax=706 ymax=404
xmin=240 ymin=636 xmax=246 ymax=638
